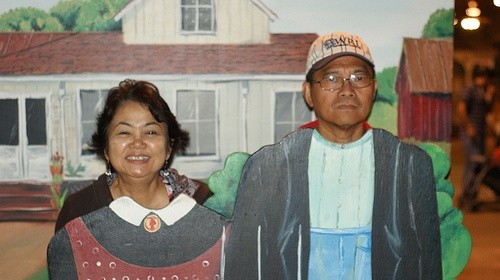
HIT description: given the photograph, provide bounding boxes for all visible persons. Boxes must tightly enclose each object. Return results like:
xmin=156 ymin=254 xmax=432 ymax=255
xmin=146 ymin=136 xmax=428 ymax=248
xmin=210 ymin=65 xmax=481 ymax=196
xmin=223 ymin=32 xmax=443 ymax=279
xmin=55 ymin=77 xmax=215 ymax=280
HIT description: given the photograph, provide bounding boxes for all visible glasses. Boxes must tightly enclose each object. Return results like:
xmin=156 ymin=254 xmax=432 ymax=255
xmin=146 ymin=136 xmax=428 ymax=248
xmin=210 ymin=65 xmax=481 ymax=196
xmin=311 ymin=74 xmax=374 ymax=91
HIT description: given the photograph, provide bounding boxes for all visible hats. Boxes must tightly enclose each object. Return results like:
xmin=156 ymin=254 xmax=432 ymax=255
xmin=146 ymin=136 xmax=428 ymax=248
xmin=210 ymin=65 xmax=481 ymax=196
xmin=305 ymin=32 xmax=375 ymax=81
